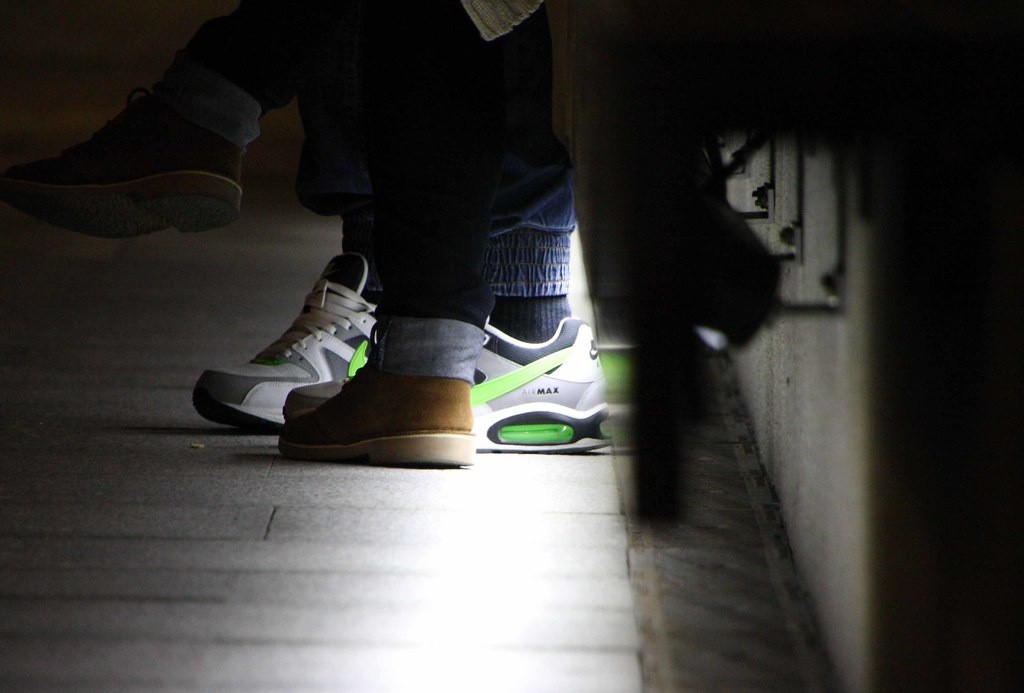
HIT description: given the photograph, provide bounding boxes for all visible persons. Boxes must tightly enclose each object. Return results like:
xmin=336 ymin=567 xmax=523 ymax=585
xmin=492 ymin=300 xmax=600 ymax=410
xmin=0 ymin=0 xmax=633 ymax=468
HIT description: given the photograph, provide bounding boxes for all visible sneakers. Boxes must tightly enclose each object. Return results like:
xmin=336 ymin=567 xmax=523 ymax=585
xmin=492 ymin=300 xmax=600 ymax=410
xmin=284 ymin=320 xmax=612 ymax=452
xmin=279 ymin=369 xmax=475 ymax=466
xmin=192 ymin=253 xmax=380 ymax=427
xmin=1 ymin=87 xmax=243 ymax=237
